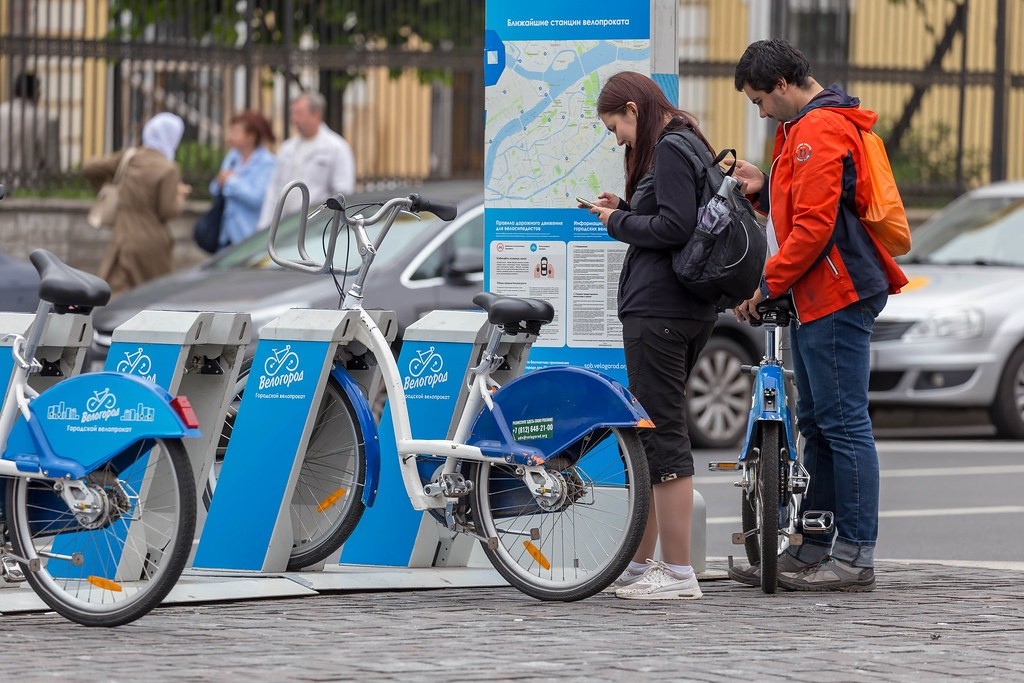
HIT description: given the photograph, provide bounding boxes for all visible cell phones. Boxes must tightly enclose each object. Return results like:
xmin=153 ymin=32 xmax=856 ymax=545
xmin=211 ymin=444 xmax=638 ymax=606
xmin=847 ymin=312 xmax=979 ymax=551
xmin=576 ymin=196 xmax=597 ymax=209
xmin=719 ymin=166 xmax=743 ymax=190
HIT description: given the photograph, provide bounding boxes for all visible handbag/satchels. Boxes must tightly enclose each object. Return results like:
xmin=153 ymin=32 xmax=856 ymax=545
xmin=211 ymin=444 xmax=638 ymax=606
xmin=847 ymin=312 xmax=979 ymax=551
xmin=192 ymin=159 xmax=236 ymax=253
xmin=87 ymin=148 xmax=136 ymax=230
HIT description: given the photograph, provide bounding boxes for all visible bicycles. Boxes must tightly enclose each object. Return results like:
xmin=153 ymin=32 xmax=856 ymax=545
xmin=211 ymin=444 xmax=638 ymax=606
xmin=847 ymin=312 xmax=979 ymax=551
xmin=0 ymin=248 xmax=384 ymax=628
xmin=708 ymin=292 xmax=835 ymax=595
xmin=203 ymin=179 xmax=657 ymax=602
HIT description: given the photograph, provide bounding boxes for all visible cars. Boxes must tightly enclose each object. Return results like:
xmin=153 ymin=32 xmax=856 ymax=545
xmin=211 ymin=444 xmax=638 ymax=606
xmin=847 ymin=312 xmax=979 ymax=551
xmin=866 ymin=180 xmax=1024 ymax=439
xmin=97 ymin=181 xmax=794 ymax=448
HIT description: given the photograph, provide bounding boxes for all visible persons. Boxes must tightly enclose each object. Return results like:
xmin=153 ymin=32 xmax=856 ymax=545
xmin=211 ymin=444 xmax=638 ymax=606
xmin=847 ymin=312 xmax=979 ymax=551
xmin=723 ymin=37 xmax=909 ymax=590
xmin=578 ymin=72 xmax=722 ymax=600
xmin=0 ymin=70 xmax=356 ymax=298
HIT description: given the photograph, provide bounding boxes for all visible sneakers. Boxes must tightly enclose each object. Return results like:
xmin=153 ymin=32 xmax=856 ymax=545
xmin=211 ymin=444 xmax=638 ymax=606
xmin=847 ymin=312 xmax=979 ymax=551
xmin=600 ymin=566 xmax=653 ymax=592
xmin=615 ymin=558 xmax=703 ymax=600
xmin=728 ymin=549 xmax=809 ymax=587
xmin=777 ymin=555 xmax=876 ymax=593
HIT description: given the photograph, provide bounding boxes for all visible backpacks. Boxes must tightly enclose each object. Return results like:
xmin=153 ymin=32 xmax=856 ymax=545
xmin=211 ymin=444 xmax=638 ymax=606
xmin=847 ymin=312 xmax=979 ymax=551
xmin=652 ymin=130 xmax=768 ymax=312
xmin=858 ymin=124 xmax=912 ymax=256
